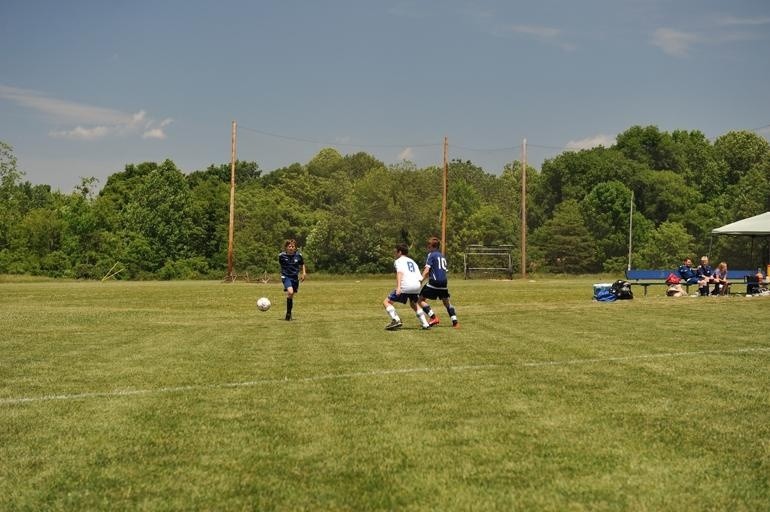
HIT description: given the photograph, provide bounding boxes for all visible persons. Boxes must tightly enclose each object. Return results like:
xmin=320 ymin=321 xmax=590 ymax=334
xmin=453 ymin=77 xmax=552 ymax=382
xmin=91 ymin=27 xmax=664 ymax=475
xmin=418 ymin=235 xmax=459 ymax=328
xmin=712 ymin=261 xmax=728 ymax=296
xmin=678 ymin=256 xmax=706 ymax=296
xmin=697 ymin=255 xmax=720 ymax=296
xmin=381 ymin=243 xmax=430 ymax=330
xmin=278 ymin=238 xmax=308 ymax=322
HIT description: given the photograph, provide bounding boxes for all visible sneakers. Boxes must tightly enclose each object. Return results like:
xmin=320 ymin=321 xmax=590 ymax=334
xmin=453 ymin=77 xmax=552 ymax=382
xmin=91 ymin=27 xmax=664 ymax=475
xmin=286 ymin=314 xmax=291 ymax=320
xmin=384 ymin=318 xmax=403 ymax=329
xmin=416 ymin=316 xmax=460 ymax=330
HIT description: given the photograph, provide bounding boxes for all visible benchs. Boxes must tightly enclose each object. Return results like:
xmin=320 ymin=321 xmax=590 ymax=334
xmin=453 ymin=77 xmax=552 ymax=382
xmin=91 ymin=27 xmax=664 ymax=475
xmin=625 ymin=270 xmax=769 ymax=296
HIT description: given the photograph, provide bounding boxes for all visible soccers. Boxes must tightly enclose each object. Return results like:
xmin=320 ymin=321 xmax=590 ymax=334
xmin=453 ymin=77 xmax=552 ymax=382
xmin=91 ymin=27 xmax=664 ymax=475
xmin=257 ymin=298 xmax=271 ymax=311
